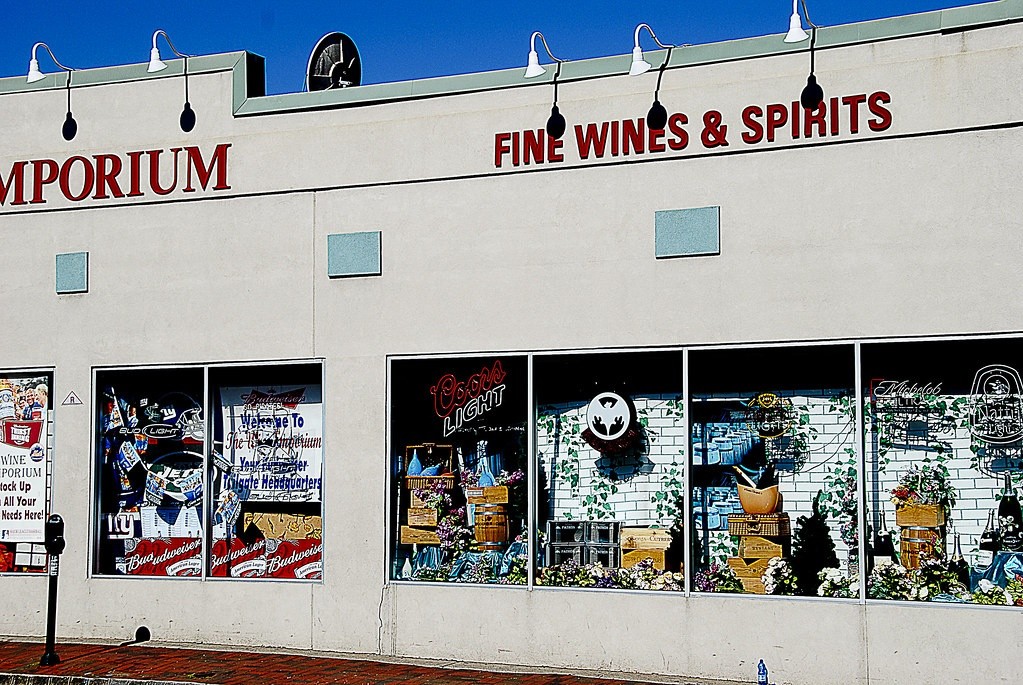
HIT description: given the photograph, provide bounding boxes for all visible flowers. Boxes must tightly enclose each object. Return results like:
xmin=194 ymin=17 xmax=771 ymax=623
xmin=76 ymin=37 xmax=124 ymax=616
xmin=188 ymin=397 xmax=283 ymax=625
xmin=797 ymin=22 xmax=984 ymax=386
xmin=412 ymin=552 xmax=1022 ymax=608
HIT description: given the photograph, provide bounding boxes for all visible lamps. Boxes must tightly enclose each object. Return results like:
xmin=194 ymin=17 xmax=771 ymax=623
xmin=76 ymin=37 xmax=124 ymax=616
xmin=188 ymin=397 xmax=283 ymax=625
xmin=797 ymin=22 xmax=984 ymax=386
xmin=147 ymin=29 xmax=194 ymax=72
xmin=27 ymin=43 xmax=73 ymax=83
xmin=523 ymin=31 xmax=565 ymax=79
xmin=783 ymin=0 xmax=820 ymax=44
xmin=628 ymin=23 xmax=674 ymax=76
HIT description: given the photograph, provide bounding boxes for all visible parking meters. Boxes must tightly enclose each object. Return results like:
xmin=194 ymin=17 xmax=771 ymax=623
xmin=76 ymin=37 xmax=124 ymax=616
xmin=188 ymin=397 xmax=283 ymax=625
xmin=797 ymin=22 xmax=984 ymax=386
xmin=39 ymin=514 xmax=65 ymax=666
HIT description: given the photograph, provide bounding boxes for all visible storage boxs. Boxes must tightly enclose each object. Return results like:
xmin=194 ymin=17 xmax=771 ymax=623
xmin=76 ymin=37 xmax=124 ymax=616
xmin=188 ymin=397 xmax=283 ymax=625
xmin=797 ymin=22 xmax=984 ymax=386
xmin=140 ymin=475 xmax=946 ymax=593
